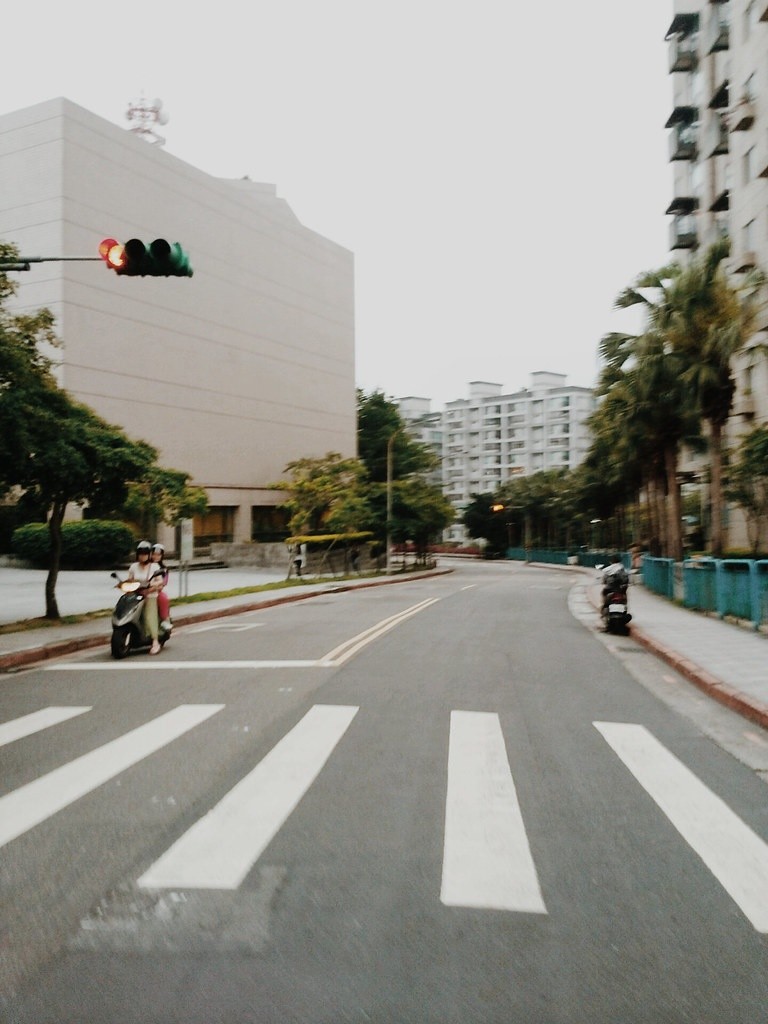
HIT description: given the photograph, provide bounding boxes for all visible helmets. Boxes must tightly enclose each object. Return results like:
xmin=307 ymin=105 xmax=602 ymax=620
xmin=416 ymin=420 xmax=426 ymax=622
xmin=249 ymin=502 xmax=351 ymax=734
xmin=137 ymin=541 xmax=152 ymax=553
xmin=152 ymin=544 xmax=165 ymax=554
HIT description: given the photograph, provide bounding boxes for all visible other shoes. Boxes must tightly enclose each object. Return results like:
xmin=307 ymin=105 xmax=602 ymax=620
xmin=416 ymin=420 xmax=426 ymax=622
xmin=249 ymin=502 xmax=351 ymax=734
xmin=150 ymin=642 xmax=161 ymax=655
xmin=162 ymin=618 xmax=172 ymax=630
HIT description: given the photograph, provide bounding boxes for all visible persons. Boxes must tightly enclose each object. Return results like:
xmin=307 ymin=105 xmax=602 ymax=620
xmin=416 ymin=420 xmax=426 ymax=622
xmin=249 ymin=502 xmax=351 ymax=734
xmin=288 ymin=543 xmax=303 ymax=578
xmin=347 ymin=545 xmax=363 ymax=576
xmin=598 ymin=535 xmax=661 ymax=619
xmin=113 ymin=540 xmax=174 ymax=655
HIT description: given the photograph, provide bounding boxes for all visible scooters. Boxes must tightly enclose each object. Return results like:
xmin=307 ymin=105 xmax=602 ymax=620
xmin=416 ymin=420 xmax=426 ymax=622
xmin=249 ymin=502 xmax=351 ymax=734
xmin=110 ymin=569 xmax=173 ymax=660
xmin=596 ymin=575 xmax=632 ymax=635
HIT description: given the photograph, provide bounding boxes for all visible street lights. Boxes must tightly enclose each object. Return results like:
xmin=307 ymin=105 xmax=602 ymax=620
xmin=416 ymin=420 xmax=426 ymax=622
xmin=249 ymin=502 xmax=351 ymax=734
xmin=387 ymin=416 xmax=443 ymax=575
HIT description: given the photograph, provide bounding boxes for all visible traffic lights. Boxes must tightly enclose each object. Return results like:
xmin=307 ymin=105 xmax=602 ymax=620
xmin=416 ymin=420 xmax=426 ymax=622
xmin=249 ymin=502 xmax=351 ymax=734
xmin=99 ymin=237 xmax=195 ymax=278
